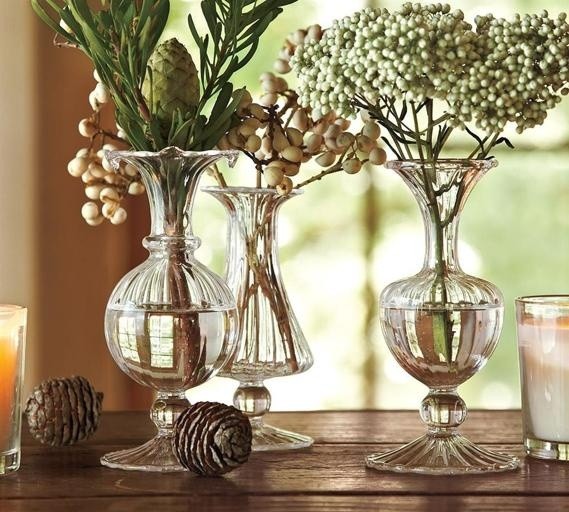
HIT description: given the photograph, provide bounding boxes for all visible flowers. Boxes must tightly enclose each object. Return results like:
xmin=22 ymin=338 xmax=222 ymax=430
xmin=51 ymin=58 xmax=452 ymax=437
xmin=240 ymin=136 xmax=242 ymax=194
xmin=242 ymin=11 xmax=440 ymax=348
xmin=53 ymin=0 xmax=568 ymax=375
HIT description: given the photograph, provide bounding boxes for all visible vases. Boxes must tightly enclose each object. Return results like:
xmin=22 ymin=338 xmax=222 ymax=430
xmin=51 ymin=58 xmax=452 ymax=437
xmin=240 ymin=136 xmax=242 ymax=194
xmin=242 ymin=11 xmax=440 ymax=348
xmin=195 ymin=183 xmax=318 ymax=456
xmin=99 ymin=150 xmax=245 ymax=471
xmin=360 ymin=154 xmax=525 ymax=471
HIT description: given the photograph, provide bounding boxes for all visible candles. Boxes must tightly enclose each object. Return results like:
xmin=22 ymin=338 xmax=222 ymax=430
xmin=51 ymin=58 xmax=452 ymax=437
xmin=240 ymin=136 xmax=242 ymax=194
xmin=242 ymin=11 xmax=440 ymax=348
xmin=517 ymin=325 xmax=569 ymax=443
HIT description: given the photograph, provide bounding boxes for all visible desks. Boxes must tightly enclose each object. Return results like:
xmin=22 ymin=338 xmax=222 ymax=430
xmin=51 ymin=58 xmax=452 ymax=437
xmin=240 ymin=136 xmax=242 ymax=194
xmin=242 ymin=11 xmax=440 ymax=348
xmin=1 ymin=403 xmax=569 ymax=512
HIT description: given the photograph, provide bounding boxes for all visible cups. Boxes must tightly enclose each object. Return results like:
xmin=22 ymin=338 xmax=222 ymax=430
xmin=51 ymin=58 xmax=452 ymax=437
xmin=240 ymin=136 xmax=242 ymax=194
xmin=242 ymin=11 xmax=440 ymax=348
xmin=0 ymin=303 xmax=27 ymax=477
xmin=514 ymin=296 xmax=569 ymax=462
